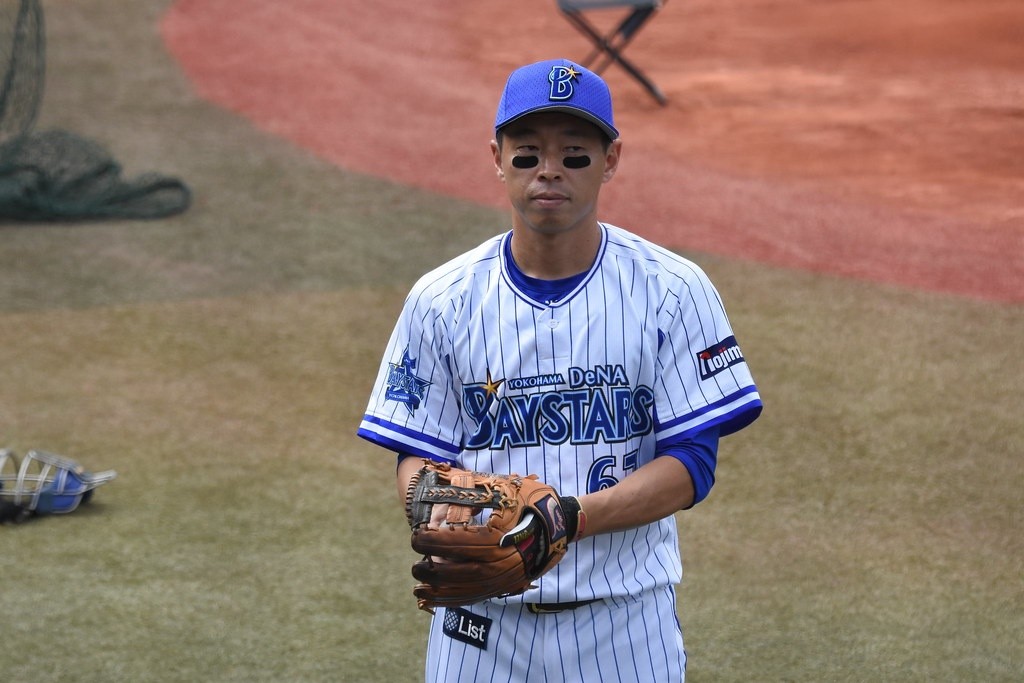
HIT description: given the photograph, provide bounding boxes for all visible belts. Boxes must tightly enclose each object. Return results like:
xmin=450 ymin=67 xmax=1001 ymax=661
xmin=522 ymin=598 xmax=604 ymax=614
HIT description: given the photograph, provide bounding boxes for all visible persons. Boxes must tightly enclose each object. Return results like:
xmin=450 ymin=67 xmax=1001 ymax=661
xmin=356 ymin=58 xmax=763 ymax=683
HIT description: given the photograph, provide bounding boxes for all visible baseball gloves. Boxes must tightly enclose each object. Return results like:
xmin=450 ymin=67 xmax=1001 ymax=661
xmin=405 ymin=456 xmax=587 ymax=615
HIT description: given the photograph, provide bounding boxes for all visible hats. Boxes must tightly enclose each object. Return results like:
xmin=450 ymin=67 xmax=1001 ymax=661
xmin=493 ymin=58 xmax=618 ymax=141
